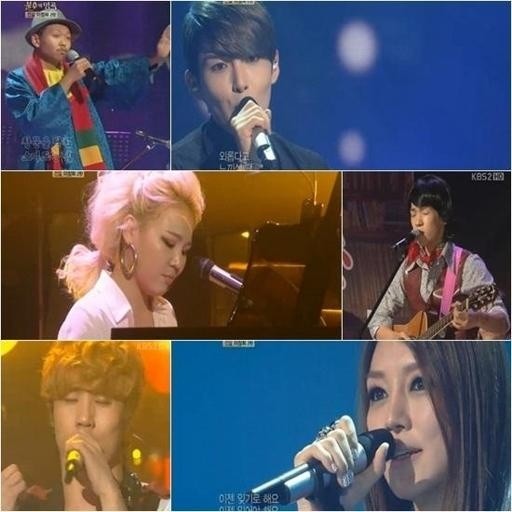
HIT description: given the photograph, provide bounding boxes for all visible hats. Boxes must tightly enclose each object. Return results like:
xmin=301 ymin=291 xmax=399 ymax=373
xmin=25 ymin=8 xmax=82 ymax=48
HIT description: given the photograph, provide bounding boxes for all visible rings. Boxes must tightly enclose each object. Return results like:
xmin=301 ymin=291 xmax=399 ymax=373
xmin=318 ymin=424 xmax=332 ymax=438
xmin=331 ymin=418 xmax=340 ymax=429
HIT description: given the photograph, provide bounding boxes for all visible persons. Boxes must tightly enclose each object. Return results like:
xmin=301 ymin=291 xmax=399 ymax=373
xmin=55 ymin=171 xmax=208 ymax=340
xmin=293 ymin=342 xmax=510 ymax=511
xmin=1 ymin=8 xmax=170 ymax=169
xmin=1 ymin=341 xmax=171 ymax=510
xmin=368 ymin=173 xmax=511 ymax=340
xmin=172 ymin=1 xmax=336 ymax=170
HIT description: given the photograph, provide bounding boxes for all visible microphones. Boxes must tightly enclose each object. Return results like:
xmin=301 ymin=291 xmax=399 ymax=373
xmin=190 ymin=257 xmax=245 ymax=295
xmin=64 ymin=449 xmax=84 ymax=483
xmin=135 ymin=129 xmax=170 ymax=150
xmin=391 ymin=229 xmax=420 ymax=250
xmin=66 ymin=49 xmax=98 ymax=83
xmin=247 ymin=429 xmax=395 ymax=508
xmin=241 ymin=97 xmax=277 ymax=170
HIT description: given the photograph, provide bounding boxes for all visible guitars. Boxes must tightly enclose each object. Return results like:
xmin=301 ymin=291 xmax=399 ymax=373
xmin=394 ymin=285 xmax=498 ymax=340
